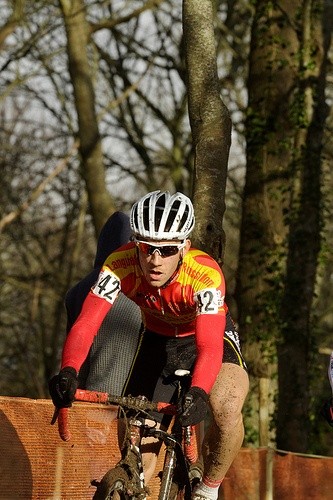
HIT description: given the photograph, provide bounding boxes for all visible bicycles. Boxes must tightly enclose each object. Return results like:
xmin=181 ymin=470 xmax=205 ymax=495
xmin=51 ymin=368 xmax=225 ymax=500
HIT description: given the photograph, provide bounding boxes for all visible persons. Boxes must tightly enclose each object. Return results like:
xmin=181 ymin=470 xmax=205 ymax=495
xmin=47 ymin=190 xmax=249 ymax=500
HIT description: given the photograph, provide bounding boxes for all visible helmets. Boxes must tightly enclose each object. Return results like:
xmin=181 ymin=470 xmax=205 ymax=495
xmin=128 ymin=190 xmax=196 ymax=241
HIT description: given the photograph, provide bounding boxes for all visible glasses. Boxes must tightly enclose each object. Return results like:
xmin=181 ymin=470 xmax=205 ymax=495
xmin=131 ymin=235 xmax=186 ymax=257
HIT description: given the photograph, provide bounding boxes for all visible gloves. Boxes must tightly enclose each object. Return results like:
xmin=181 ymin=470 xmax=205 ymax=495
xmin=48 ymin=367 xmax=79 ymax=409
xmin=175 ymin=387 xmax=210 ymax=429
